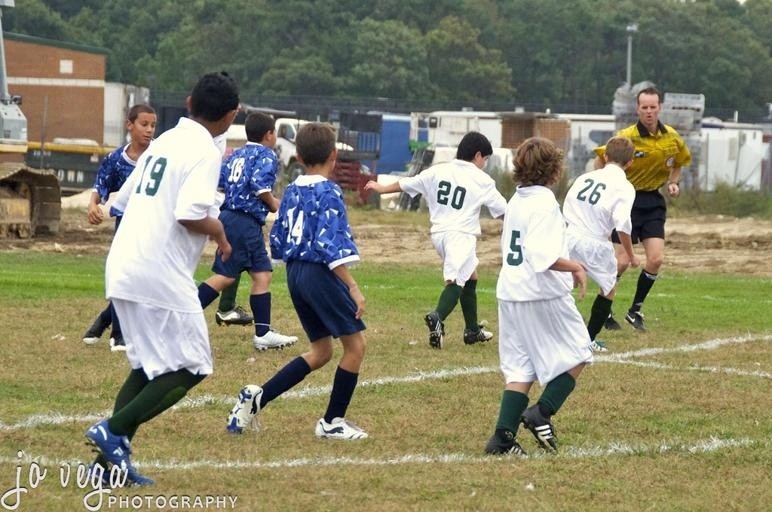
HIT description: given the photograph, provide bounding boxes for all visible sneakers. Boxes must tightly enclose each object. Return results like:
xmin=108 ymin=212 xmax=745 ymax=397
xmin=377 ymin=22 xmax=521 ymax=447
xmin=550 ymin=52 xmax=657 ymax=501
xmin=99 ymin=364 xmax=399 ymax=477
xmin=253 ymin=331 xmax=299 ymax=352
xmin=79 ymin=419 xmax=156 ymax=491
xmin=82 ymin=313 xmax=109 ymax=348
xmin=313 ymin=417 xmax=368 ymax=442
xmin=108 ymin=328 xmax=128 ymax=351
xmin=604 ymin=311 xmax=622 ymax=333
xmin=462 ymin=327 xmax=494 ymax=346
xmin=214 ymin=306 xmax=254 ymax=327
xmin=423 ymin=310 xmax=444 ymax=349
xmin=520 ymin=403 xmax=560 ymax=457
xmin=485 ymin=428 xmax=527 ymax=460
xmin=624 ymin=311 xmax=648 ymax=332
xmin=590 ymin=338 xmax=610 ymax=358
xmin=225 ymin=383 xmax=264 ymax=437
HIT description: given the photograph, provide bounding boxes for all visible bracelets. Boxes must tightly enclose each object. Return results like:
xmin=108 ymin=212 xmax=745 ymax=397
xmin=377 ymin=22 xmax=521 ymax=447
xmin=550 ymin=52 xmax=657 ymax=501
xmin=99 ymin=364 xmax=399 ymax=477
xmin=668 ymin=182 xmax=679 ymax=186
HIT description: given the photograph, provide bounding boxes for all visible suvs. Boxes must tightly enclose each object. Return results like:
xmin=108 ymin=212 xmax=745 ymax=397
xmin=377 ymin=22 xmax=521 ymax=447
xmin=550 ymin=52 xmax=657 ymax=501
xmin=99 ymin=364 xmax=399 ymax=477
xmin=271 ymin=113 xmax=355 ymax=184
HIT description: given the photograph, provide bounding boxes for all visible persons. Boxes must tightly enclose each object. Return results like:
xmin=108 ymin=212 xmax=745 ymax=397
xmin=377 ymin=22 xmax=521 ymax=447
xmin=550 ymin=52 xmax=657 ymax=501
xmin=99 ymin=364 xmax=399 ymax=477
xmin=485 ymin=138 xmax=595 ymax=456
xmin=226 ymin=123 xmax=369 ymax=440
xmin=562 ymin=138 xmax=641 ymax=350
xmin=83 ymin=104 xmax=157 ymax=351
xmin=593 ymin=86 xmax=692 ymax=331
xmin=362 ymin=131 xmax=508 ymax=349
xmin=84 ymin=70 xmax=239 ymax=488
xmin=216 ymin=275 xmax=255 ymax=323
xmin=196 ymin=113 xmax=299 ymax=351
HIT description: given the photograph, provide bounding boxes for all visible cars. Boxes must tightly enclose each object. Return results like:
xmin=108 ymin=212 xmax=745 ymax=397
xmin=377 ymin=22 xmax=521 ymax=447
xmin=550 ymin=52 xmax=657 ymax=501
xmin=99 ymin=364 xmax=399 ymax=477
xmin=700 ymin=115 xmax=725 ymax=129
xmin=223 ymin=106 xmax=298 ymax=142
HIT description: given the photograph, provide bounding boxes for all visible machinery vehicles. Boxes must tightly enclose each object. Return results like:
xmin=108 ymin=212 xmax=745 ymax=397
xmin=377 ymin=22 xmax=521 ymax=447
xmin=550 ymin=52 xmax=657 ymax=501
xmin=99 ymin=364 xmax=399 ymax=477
xmin=0 ymin=0 xmax=66 ymax=249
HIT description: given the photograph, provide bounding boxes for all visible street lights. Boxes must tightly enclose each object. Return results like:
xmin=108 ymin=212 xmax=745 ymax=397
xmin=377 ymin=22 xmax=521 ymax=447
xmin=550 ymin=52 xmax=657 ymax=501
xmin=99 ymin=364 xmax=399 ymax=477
xmin=624 ymin=20 xmax=641 ymax=92
